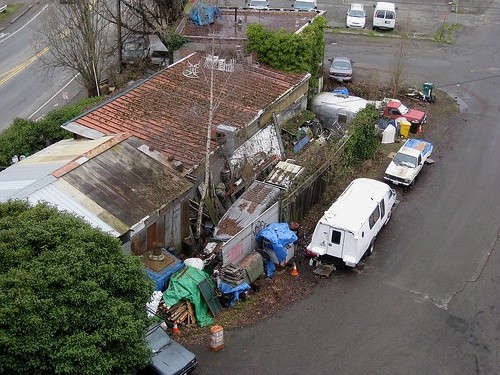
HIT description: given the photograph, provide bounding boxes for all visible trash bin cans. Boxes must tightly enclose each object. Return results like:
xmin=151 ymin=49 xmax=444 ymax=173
xmin=423 ymin=82 xmax=433 ymax=96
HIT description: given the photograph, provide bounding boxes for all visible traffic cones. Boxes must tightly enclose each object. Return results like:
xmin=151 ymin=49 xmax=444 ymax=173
xmin=172 ymin=321 xmax=178 ymax=333
xmin=291 ymin=262 xmax=299 ymax=275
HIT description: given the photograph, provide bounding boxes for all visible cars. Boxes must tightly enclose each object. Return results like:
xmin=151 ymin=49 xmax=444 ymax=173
xmin=384 ymin=102 xmax=426 ymax=124
xmin=122 ymin=42 xmax=169 ymax=65
xmin=247 ymin=0 xmax=269 ymax=11
xmin=328 ymin=55 xmax=355 ymax=82
xmin=142 ymin=325 xmax=199 ymax=375
xmin=345 ymin=3 xmax=367 ymax=28
xmin=383 ymin=138 xmax=433 ymax=186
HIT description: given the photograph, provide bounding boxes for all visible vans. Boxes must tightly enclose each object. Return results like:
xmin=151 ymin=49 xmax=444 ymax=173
xmin=372 ymin=2 xmax=398 ymax=31
xmin=291 ymin=0 xmax=317 ymax=12
xmin=306 ymin=178 xmax=396 ymax=267
